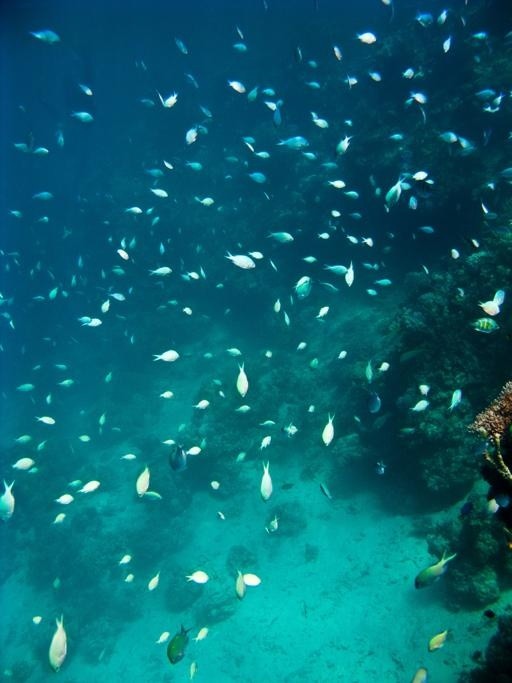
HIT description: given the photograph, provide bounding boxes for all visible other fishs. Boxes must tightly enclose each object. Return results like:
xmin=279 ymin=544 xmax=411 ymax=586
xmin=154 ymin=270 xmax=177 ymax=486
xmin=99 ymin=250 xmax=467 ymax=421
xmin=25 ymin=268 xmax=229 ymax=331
xmin=1 ymin=0 xmax=510 ymax=682
